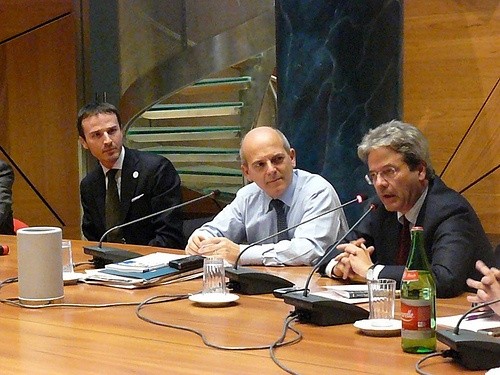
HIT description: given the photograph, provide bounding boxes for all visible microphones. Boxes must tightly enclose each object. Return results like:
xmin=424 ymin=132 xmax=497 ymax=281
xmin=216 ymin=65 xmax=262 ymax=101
xmin=82 ymin=190 xmax=221 ymax=268
xmin=224 ymin=193 xmax=368 ymax=295
xmin=280 ymin=196 xmax=383 ymax=326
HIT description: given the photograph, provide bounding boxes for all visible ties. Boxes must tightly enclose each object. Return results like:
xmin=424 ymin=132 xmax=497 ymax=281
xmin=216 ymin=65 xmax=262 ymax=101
xmin=266 ymin=199 xmax=288 ymax=242
xmin=105 ymin=169 xmax=124 ymax=243
xmin=395 ymin=214 xmax=411 ymax=266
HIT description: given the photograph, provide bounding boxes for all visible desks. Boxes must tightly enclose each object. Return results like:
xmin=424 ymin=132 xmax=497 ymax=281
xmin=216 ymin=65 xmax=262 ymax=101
xmin=0 ymin=236 xmax=500 ymax=375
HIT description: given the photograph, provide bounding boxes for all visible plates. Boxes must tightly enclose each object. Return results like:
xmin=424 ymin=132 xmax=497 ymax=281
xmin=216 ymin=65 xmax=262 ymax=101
xmin=353 ymin=319 xmax=403 ymax=336
xmin=190 ymin=293 xmax=240 ymax=308
xmin=60 ymin=272 xmax=84 ymax=285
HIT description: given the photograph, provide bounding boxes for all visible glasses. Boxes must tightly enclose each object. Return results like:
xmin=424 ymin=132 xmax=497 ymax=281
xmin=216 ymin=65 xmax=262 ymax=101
xmin=364 ymin=157 xmax=408 ymax=185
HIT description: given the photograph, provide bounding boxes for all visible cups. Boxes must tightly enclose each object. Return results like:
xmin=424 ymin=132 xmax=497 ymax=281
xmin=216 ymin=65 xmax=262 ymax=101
xmin=202 ymin=255 xmax=228 ymax=301
xmin=60 ymin=240 xmax=74 ymax=276
xmin=368 ymin=280 xmax=396 ymax=328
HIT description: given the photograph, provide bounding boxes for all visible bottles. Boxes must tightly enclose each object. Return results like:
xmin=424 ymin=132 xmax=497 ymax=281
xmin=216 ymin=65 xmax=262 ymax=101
xmin=400 ymin=226 xmax=438 ymax=354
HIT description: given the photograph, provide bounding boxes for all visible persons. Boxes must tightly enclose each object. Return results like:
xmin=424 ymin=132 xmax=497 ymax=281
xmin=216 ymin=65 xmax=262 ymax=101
xmin=0 ymin=160 xmax=15 ymax=234
xmin=77 ymin=101 xmax=185 ymax=249
xmin=316 ymin=120 xmax=493 ymax=298
xmin=466 ymin=243 xmax=500 ymax=316
xmin=185 ymin=126 xmax=349 ymax=268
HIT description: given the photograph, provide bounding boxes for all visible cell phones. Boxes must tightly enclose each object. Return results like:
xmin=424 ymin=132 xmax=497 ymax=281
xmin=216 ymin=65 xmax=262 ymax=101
xmin=169 ymin=254 xmax=205 ymax=270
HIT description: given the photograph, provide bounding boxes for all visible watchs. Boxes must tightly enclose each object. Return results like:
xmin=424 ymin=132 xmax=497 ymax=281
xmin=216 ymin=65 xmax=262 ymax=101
xmin=366 ymin=264 xmax=377 ymax=282
